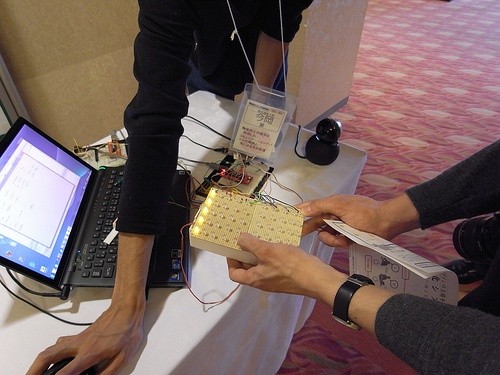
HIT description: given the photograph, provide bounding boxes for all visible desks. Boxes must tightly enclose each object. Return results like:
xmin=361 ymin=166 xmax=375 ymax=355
xmin=0 ymin=92 xmax=367 ymax=375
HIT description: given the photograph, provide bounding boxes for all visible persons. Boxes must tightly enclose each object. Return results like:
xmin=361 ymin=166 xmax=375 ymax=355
xmin=25 ymin=0 xmax=313 ymax=375
xmin=226 ymin=140 xmax=500 ymax=375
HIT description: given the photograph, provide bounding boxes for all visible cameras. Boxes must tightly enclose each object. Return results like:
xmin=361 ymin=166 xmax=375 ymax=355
xmin=453 ymin=210 xmax=500 ymax=266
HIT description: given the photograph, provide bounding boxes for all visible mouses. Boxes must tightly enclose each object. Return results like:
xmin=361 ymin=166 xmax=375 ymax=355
xmin=41 ymin=356 xmax=101 ymax=375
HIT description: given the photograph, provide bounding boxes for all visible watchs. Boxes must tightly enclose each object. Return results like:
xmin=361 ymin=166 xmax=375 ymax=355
xmin=331 ymin=274 xmax=375 ymax=332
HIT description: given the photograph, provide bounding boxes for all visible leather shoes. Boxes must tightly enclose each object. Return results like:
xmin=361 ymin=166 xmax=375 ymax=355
xmin=441 ymin=257 xmax=484 ymax=284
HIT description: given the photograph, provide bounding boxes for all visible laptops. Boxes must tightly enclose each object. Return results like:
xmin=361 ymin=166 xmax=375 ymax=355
xmin=0 ymin=117 xmax=190 ymax=289
